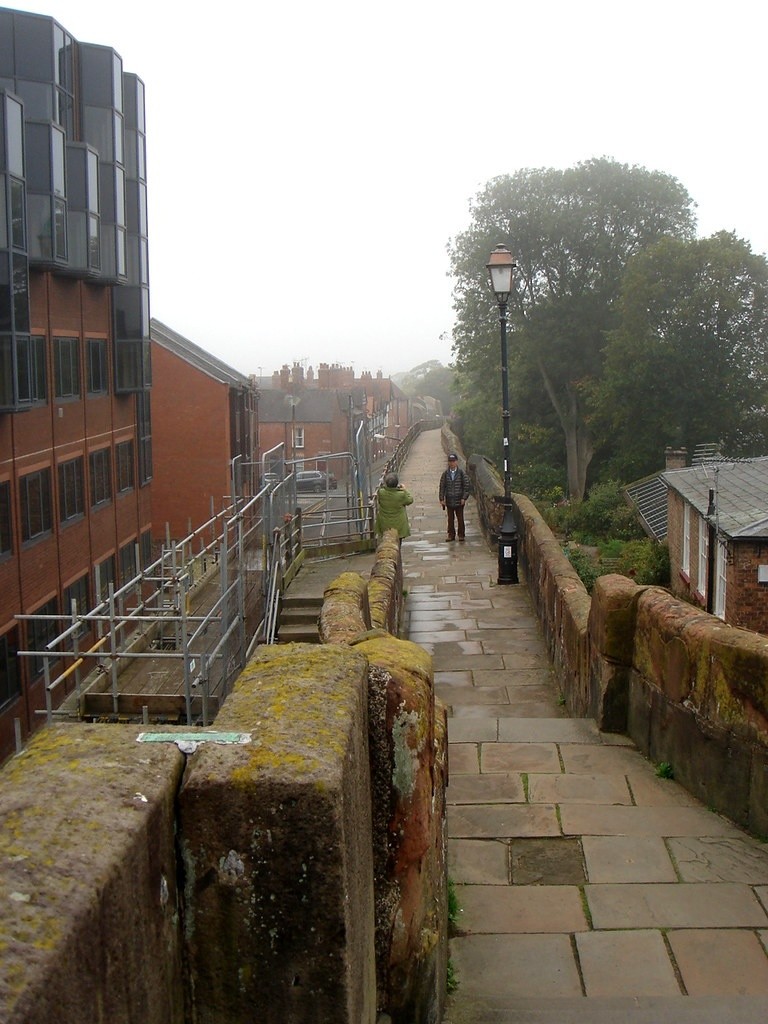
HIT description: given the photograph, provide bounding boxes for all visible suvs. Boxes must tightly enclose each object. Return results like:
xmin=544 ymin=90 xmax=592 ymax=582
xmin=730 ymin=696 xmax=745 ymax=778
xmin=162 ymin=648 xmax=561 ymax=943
xmin=296 ymin=470 xmax=331 ymax=494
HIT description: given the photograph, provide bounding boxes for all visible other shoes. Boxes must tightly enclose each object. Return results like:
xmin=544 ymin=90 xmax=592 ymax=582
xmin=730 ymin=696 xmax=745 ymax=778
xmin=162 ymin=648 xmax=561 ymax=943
xmin=445 ymin=534 xmax=455 ymax=541
xmin=459 ymin=536 xmax=464 ymax=540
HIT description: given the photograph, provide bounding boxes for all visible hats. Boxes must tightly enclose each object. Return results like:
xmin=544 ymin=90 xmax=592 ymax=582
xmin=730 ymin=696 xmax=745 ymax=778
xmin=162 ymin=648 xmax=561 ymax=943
xmin=448 ymin=454 xmax=458 ymax=461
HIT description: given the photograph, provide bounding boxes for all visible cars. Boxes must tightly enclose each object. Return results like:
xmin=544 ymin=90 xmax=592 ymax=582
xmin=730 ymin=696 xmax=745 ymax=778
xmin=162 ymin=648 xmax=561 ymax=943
xmin=264 ymin=473 xmax=279 ymax=487
xmin=325 ymin=472 xmax=338 ymax=489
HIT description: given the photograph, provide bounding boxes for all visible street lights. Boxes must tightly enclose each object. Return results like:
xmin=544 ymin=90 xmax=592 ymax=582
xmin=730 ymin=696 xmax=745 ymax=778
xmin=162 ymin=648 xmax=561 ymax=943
xmin=258 ymin=366 xmax=262 ymax=386
xmin=482 ymin=242 xmax=519 ymax=585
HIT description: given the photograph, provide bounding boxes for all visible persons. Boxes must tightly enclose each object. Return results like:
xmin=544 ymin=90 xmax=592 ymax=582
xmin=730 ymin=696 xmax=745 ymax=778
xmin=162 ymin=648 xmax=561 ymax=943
xmin=439 ymin=454 xmax=470 ymax=542
xmin=377 ymin=472 xmax=413 ymax=547
